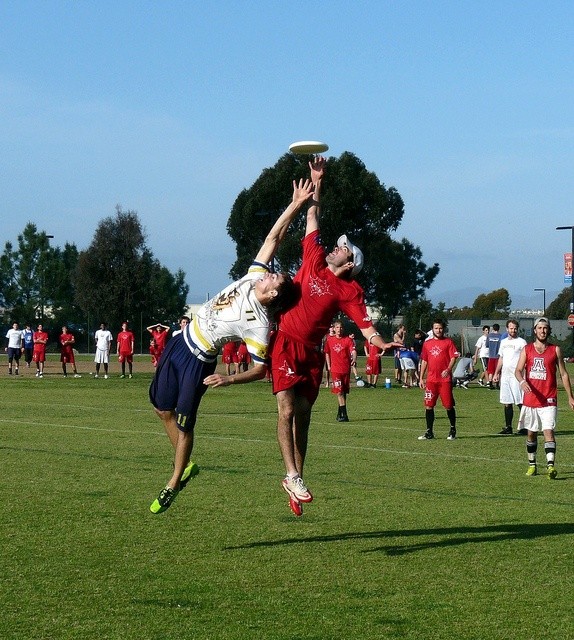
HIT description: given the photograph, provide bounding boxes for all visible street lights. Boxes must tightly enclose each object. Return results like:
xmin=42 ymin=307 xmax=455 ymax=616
xmin=535 ymin=288 xmax=546 ymax=317
xmin=556 ymin=226 xmax=574 ymax=363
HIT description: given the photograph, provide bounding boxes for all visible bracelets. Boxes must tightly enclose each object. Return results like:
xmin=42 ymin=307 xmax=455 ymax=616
xmin=367 ymin=331 xmax=382 ymax=347
xmin=520 ymin=379 xmax=526 ymax=387
xmin=446 ymin=367 xmax=451 ymax=374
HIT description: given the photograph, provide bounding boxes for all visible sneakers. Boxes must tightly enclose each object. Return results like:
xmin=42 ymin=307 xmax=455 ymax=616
xmin=26 ymin=363 xmax=30 ymax=367
xmin=325 ymin=382 xmax=329 ymax=387
xmin=336 ymin=414 xmax=342 ymax=422
xmin=456 ymin=382 xmax=460 ymax=388
xmin=495 ymin=387 xmax=500 ymax=390
xmin=355 ymin=375 xmax=362 ymax=379
xmin=417 ymin=431 xmax=434 ymax=440
xmin=120 ymin=374 xmax=125 ymax=378
xmin=369 ymin=384 xmax=376 ymax=388
xmin=340 ymin=415 xmax=349 ymax=422
xmin=178 ymin=463 xmax=200 ymax=492
xmin=128 ymin=373 xmax=133 ymax=379
xmin=15 ymin=369 xmax=18 ymax=374
xmin=395 ymin=379 xmax=397 ymax=383
xmin=401 ymin=383 xmax=408 ymax=388
xmin=104 ymin=374 xmax=108 ymax=379
xmin=230 ymin=370 xmax=236 ymax=374
xmin=526 ymin=464 xmax=537 ymax=476
xmin=460 ymin=383 xmax=468 ymax=389
xmin=446 ymin=430 xmax=456 ymax=440
xmin=282 ymin=471 xmax=313 ymax=503
xmin=150 ymin=482 xmax=181 ymax=513
xmin=396 ymin=380 xmax=402 ymax=384
xmin=266 ymin=379 xmax=271 ymax=382
xmin=63 ymin=373 xmax=67 ymax=378
xmin=516 ymin=429 xmax=528 ymax=435
xmin=39 ymin=371 xmax=43 ymax=378
xmin=35 ymin=369 xmax=39 ymax=376
xmin=486 ymin=382 xmax=490 ymax=387
xmin=94 ymin=375 xmax=99 ymax=378
xmin=9 ymin=368 xmax=12 ymax=374
xmin=546 ymin=465 xmax=558 ymax=480
xmin=73 ymin=374 xmax=82 ymax=378
xmin=476 ymin=379 xmax=485 ymax=386
xmin=487 ymin=386 xmax=495 ymax=390
xmin=364 ymin=383 xmax=371 ymax=387
xmin=410 ymin=384 xmax=413 ymax=388
xmin=497 ymin=425 xmax=513 ymax=434
xmin=288 ymin=496 xmax=303 ymax=516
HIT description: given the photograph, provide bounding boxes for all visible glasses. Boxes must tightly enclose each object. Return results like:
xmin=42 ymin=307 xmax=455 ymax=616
xmin=330 ymin=326 xmax=334 ymax=328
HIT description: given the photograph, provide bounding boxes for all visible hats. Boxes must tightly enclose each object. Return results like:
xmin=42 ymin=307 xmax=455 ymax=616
xmin=118 ymin=354 xmax=124 ymax=362
xmin=533 ymin=317 xmax=550 ymax=327
xmin=337 ymin=234 xmax=364 ymax=279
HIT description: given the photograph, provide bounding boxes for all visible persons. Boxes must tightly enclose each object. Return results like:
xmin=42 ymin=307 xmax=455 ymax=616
xmin=323 ymin=319 xmax=358 ymax=423
xmin=270 ymin=152 xmax=406 ymax=517
xmin=171 ymin=316 xmax=191 ymax=338
xmin=405 ymin=347 xmax=410 ymax=354
xmin=116 ymin=321 xmax=135 ymax=379
xmin=392 ymin=322 xmax=406 ymax=384
xmin=22 ymin=322 xmax=35 ymax=369
xmin=485 ymin=323 xmax=503 ymax=391
xmin=220 ymin=338 xmax=240 ymax=376
xmin=148 ymin=177 xmax=317 ymax=515
xmin=410 ymin=345 xmax=416 ymax=353
xmin=93 ymin=321 xmax=114 ymax=379
xmin=264 ymin=326 xmax=276 ymax=384
xmin=345 ymin=331 xmax=363 ymax=381
xmin=364 ymin=325 xmax=387 ymax=389
xmin=3 ymin=322 xmax=25 ymax=375
xmin=415 ymin=318 xmax=460 ymax=442
xmin=342 ymin=319 xmax=344 ymax=321
xmin=146 ymin=322 xmax=171 ymax=350
xmin=320 ymin=322 xmax=335 ymax=390
xmin=514 ymin=315 xmax=573 ymax=482
xmin=230 ymin=339 xmax=253 ymax=376
xmin=452 ymin=351 xmax=479 ymax=391
xmin=273 ymin=330 xmax=278 ymax=338
xmin=500 ymin=320 xmax=509 ymax=347
xmin=567 ymin=356 xmax=574 ymax=363
xmin=398 ymin=350 xmax=426 ymax=390
xmin=492 ymin=318 xmax=529 ymax=435
xmin=473 ymin=324 xmax=490 ymax=390
xmin=412 ymin=329 xmax=426 ymax=388
xmin=57 ymin=324 xmax=82 ymax=378
xmin=149 ymin=337 xmax=165 ymax=372
xmin=562 ymin=355 xmax=567 ymax=363
xmin=32 ymin=323 xmax=49 ymax=378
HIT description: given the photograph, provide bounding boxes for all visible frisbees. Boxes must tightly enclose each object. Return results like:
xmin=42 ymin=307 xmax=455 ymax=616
xmin=291 ymin=141 xmax=329 ymax=154
